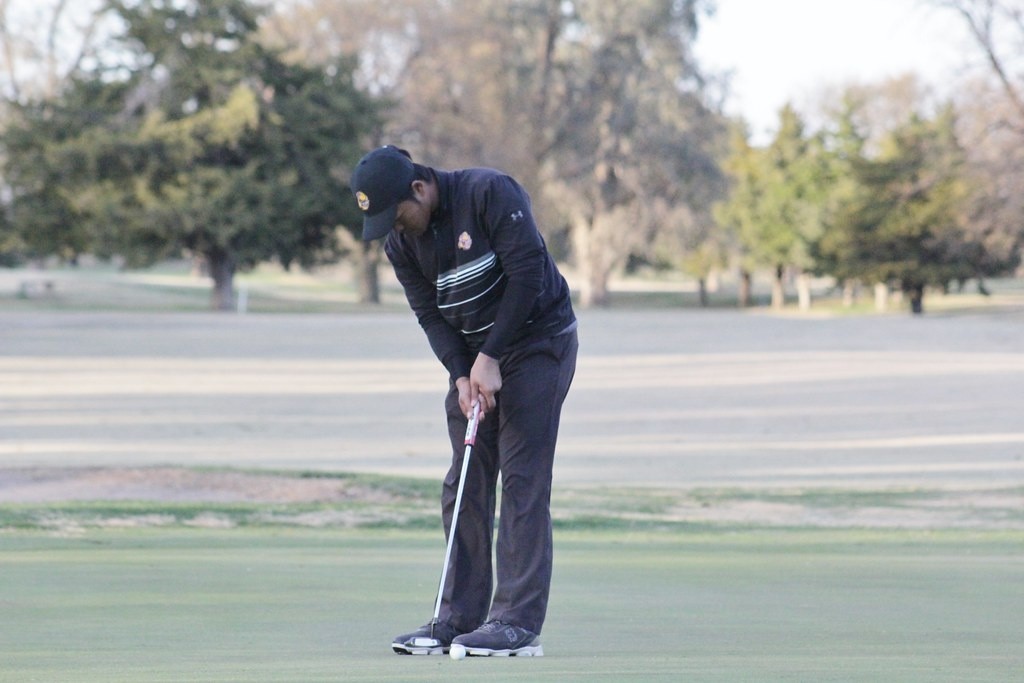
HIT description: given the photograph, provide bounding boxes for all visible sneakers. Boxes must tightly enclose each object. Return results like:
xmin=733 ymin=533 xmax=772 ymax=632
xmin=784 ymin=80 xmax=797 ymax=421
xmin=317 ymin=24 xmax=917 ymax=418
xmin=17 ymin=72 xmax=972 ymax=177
xmin=450 ymin=619 xmax=545 ymax=658
xmin=391 ymin=618 xmax=464 ymax=654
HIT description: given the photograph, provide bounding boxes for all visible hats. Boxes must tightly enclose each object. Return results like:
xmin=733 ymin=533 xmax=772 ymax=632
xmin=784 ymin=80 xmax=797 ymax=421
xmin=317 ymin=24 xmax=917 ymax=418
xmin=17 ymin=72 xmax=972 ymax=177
xmin=354 ymin=144 xmax=413 ymax=240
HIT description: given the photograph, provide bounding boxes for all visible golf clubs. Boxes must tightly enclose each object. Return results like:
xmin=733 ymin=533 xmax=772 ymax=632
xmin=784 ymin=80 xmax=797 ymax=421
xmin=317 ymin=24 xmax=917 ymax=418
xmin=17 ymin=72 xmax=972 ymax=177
xmin=404 ymin=401 xmax=487 ymax=648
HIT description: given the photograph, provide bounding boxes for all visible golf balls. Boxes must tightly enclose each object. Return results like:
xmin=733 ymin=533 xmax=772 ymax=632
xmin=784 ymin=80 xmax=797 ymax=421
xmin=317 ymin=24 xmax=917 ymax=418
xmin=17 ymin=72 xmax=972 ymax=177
xmin=448 ymin=643 xmax=467 ymax=661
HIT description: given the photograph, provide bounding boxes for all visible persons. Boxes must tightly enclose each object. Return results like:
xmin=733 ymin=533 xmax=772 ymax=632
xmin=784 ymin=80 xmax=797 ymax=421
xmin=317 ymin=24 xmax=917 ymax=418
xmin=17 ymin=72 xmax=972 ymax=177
xmin=350 ymin=146 xmax=579 ymax=659
